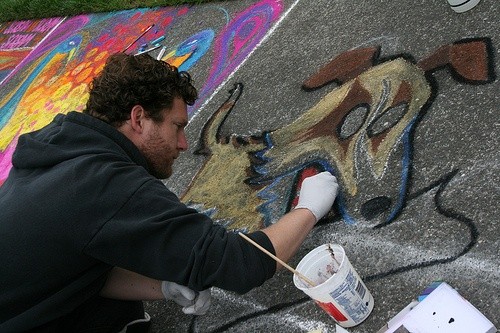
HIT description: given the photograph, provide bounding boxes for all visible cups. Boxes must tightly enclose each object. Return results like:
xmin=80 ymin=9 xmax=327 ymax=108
xmin=448 ymin=0 xmax=481 ymax=13
xmin=293 ymin=243 xmax=374 ymax=328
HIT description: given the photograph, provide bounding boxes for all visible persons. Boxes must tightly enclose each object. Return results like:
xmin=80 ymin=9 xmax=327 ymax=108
xmin=0 ymin=52 xmax=339 ymax=333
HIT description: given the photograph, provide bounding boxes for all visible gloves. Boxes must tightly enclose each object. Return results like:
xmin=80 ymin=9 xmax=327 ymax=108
xmin=294 ymin=170 xmax=339 ymax=222
xmin=161 ymin=280 xmax=212 ymax=316
